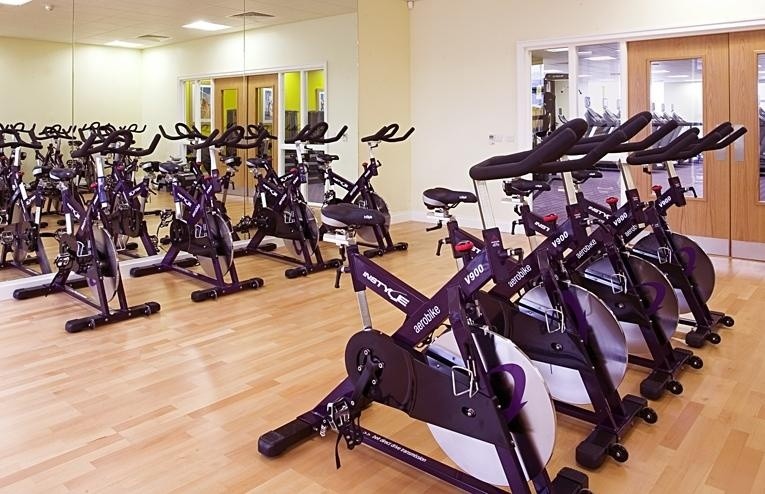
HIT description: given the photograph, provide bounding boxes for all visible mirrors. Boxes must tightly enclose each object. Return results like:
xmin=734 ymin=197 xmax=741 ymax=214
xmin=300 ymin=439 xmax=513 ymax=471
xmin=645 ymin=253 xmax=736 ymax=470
xmin=0 ymin=0 xmax=357 ymax=283
